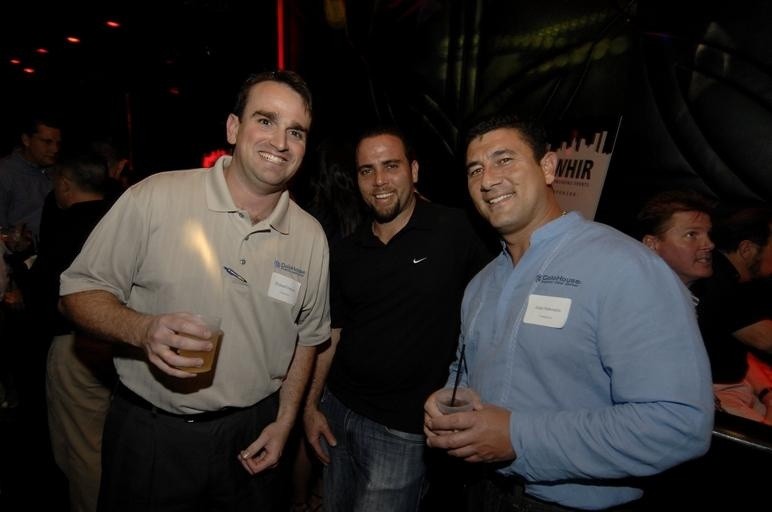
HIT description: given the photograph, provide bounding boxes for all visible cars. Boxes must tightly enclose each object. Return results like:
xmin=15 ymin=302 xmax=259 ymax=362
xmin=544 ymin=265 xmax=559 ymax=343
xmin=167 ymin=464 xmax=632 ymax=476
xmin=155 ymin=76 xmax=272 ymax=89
xmin=434 ymin=387 xmax=474 ymax=416
xmin=177 ymin=324 xmax=219 ymax=372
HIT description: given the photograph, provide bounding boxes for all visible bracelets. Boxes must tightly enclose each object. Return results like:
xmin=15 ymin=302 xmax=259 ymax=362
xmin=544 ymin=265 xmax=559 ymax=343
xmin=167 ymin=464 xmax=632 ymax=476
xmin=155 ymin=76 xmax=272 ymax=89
xmin=757 ymin=386 xmax=769 ymax=404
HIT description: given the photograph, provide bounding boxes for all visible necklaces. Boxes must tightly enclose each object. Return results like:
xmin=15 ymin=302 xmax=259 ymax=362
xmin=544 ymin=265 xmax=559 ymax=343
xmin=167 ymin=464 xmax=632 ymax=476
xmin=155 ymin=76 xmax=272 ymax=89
xmin=560 ymin=209 xmax=567 ymax=217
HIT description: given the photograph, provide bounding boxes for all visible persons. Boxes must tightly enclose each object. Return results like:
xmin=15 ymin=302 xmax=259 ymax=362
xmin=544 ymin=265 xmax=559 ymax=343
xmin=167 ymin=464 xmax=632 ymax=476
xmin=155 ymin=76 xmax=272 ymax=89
xmin=55 ymin=68 xmax=334 ymax=511
xmin=636 ymin=186 xmax=769 ymax=407
xmin=302 ymin=118 xmax=501 ymax=510
xmin=424 ymin=112 xmax=714 ymax=511
xmin=688 ymin=207 xmax=768 ymax=383
xmin=4 ymin=110 xmax=131 ymax=509
